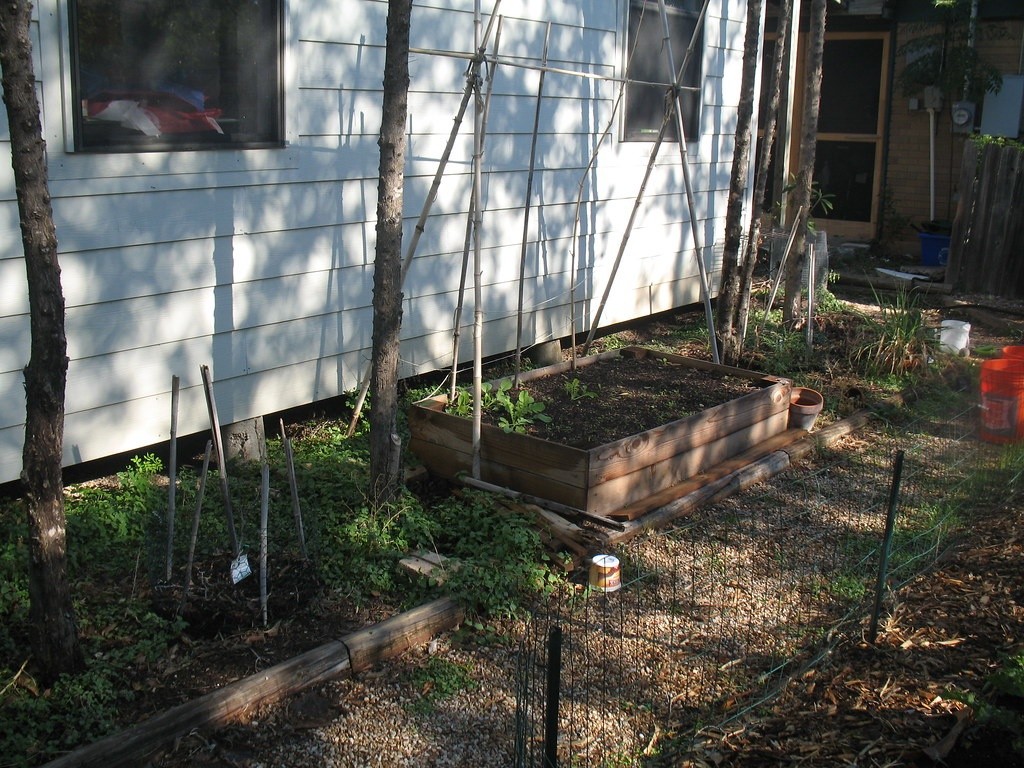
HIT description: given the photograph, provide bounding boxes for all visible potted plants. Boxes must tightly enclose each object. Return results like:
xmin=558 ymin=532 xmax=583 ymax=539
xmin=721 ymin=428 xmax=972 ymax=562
xmin=407 ymin=347 xmax=790 ymax=522
xmin=850 ymin=270 xmax=949 ymax=373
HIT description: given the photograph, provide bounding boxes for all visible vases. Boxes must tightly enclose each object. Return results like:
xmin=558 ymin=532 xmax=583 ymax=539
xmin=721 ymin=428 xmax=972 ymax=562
xmin=789 ymin=387 xmax=823 ymax=431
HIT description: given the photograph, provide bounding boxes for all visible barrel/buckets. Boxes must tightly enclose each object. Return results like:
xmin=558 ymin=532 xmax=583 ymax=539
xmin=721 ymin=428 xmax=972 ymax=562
xmin=919 ymin=232 xmax=951 ymax=265
xmin=940 ymin=320 xmax=971 ymax=355
xmin=1002 ymin=346 xmax=1024 ymax=358
xmin=980 ymin=358 xmax=1024 ymax=443
xmin=586 ymin=555 xmax=621 ymax=592
